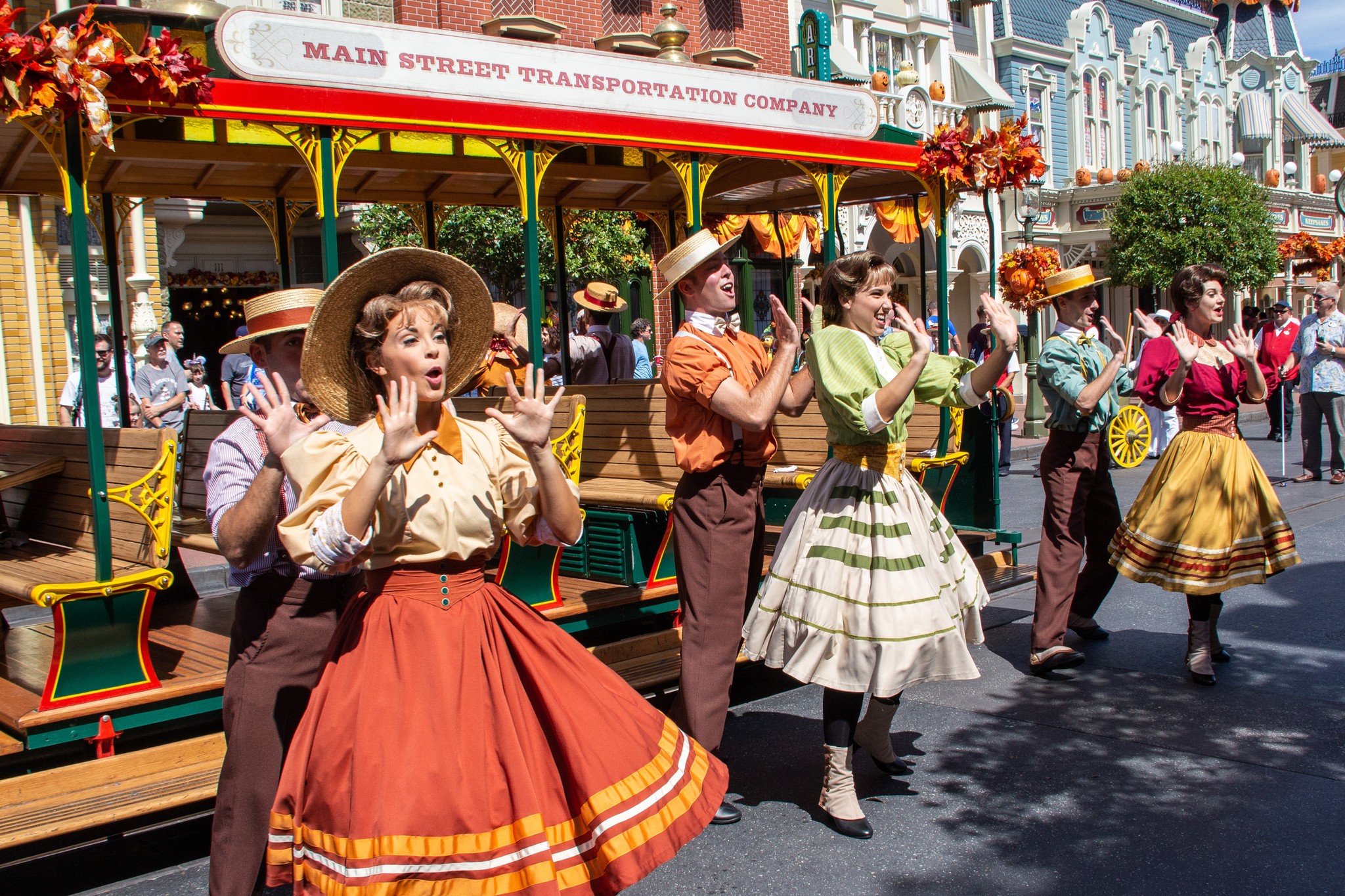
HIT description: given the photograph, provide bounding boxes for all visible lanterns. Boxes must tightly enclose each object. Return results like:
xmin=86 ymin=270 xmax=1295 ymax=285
xmin=929 ymin=82 xmax=945 ymax=101
xmin=872 ymin=71 xmax=888 ymax=92
xmin=1075 ymin=161 xmax=1150 ymax=186
xmin=1315 ymin=174 xmax=1326 ymax=194
xmin=1264 ymin=170 xmax=1280 ymax=188
xmin=895 ymin=60 xmax=920 ymax=87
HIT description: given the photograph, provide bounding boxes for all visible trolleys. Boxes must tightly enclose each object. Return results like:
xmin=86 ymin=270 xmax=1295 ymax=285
xmin=1104 ymin=396 xmax=1155 ymax=468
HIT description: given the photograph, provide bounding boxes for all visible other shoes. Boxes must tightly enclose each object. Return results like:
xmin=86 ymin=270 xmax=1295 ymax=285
xmin=1033 ymin=468 xmax=1041 ymax=477
xmin=999 ymin=469 xmax=1009 ymax=476
xmin=997 ymin=417 xmax=1018 ymax=431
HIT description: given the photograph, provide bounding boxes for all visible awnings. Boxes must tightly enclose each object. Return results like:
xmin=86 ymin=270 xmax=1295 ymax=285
xmin=829 ymin=33 xmax=872 ymax=86
xmin=1236 ymin=93 xmax=1345 ymax=156
xmin=950 ymin=55 xmax=1015 ymax=115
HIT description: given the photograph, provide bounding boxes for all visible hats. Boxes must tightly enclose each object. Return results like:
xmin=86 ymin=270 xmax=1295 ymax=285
xmin=926 ymin=322 xmax=953 ymax=340
xmin=652 ymin=228 xmax=741 ymax=301
xmin=1033 ymin=264 xmax=1111 ymax=303
xmin=1272 ymin=300 xmax=1290 ymax=310
xmin=1147 ymin=309 xmax=1172 ymax=323
xmin=218 ymin=288 xmax=325 ymax=357
xmin=980 ymin=321 xmax=991 ymax=335
xmin=236 ymin=326 xmax=248 ymax=337
xmin=486 ymin=302 xmax=528 ymax=367
xmin=577 ymin=309 xmax=584 ymax=320
xmin=144 ymin=333 xmax=169 ymax=349
xmin=299 ymin=246 xmax=495 ymax=426
xmin=574 ymin=282 xmax=628 ymax=313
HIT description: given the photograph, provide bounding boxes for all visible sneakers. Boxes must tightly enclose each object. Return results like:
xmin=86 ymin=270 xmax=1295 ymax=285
xmin=1267 ymin=430 xmax=1292 ymax=441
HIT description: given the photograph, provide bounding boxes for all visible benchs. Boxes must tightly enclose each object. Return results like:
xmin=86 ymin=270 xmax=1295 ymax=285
xmin=485 ymin=379 xmax=678 ymax=590
xmin=760 ymin=395 xmax=840 ymax=529
xmin=905 ymin=398 xmax=972 ymax=516
xmin=0 ymin=421 xmax=187 ymax=712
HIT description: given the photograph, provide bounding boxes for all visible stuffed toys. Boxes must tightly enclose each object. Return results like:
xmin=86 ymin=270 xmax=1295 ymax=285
xmin=759 ymin=322 xmax=776 ymax=361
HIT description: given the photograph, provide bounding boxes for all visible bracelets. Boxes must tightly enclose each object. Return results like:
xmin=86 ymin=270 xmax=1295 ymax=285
xmin=158 ymin=423 xmax=165 ymax=428
xmin=240 ymin=395 xmax=246 ymax=398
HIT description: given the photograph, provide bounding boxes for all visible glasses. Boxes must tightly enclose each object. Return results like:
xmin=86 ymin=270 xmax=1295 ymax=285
xmin=1312 ymin=293 xmax=1335 ymax=300
xmin=95 ymin=348 xmax=111 ymax=357
xmin=802 ymin=338 xmax=809 ymax=343
xmin=642 ymin=328 xmax=652 ymax=332
xmin=123 ymin=335 xmax=128 ymax=340
xmin=1273 ymin=309 xmax=1289 ymax=314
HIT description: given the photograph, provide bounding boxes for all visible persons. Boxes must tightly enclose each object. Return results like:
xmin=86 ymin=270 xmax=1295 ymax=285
xmin=1277 ymin=281 xmax=1345 ymax=484
xmin=1128 ymin=309 xmax=1180 ymax=459
xmin=59 ymin=321 xmax=268 ymax=500
xmin=1108 ymin=264 xmax=1301 ymax=684
xmin=1241 ymin=306 xmax=1301 ymax=441
xmin=741 ymin=250 xmax=1018 ymax=839
xmin=652 ymin=228 xmax=814 ymax=824
xmin=266 ymin=246 xmax=730 ymax=896
xmin=793 ymin=328 xmax=811 ymax=372
xmin=876 ymin=300 xmax=1019 ymax=434
xmin=202 ymin=289 xmax=457 ymax=896
xmin=453 ymin=282 xmax=663 ymax=397
xmin=977 ymin=321 xmax=1020 ymax=476
xmin=1085 ymin=324 xmax=1099 ymax=340
xmin=1029 ymin=264 xmax=1139 ymax=672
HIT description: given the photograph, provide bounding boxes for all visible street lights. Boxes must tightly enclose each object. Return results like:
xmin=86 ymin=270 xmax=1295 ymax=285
xmin=1007 ymin=175 xmax=1060 ymax=439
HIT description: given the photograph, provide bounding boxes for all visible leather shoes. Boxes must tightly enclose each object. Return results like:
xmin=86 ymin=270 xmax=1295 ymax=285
xmin=1292 ymin=474 xmax=1314 ymax=482
xmin=709 ymin=800 xmax=742 ymax=825
xmin=1331 ymin=472 xmax=1344 ymax=484
xmin=1028 ymin=606 xmax=1109 ymax=673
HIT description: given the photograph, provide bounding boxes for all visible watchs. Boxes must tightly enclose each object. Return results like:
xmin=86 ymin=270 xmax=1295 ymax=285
xmin=1328 ymin=345 xmax=1336 ymax=356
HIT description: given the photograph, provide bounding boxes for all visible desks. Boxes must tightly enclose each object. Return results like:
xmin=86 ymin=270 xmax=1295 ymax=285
xmin=170 ymin=394 xmax=587 ymax=616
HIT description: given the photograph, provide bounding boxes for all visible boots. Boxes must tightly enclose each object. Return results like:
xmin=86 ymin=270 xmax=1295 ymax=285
xmin=852 ymin=693 xmax=908 ymax=775
xmin=814 ymin=744 xmax=873 ymax=839
xmin=1184 ymin=599 xmax=1231 ymax=685
